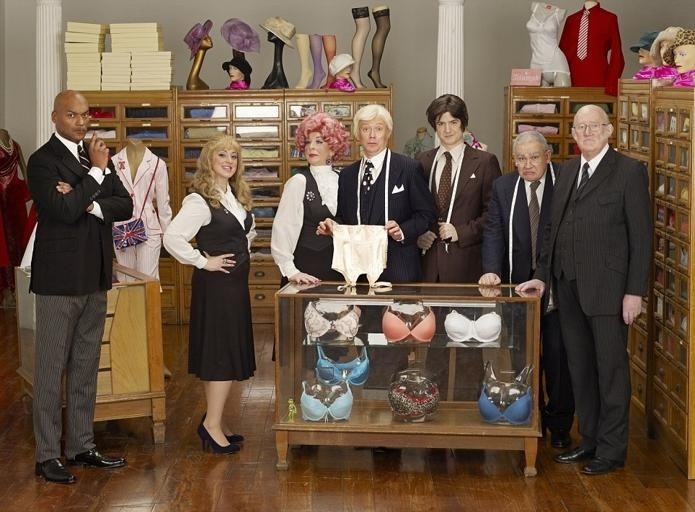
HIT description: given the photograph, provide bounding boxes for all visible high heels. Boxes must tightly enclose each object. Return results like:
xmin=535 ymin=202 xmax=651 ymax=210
xmin=197 ymin=422 xmax=240 ymax=454
xmin=202 ymin=412 xmax=243 ymax=443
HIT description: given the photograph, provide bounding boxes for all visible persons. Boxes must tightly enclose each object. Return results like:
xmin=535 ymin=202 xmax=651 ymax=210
xmin=559 ymin=1 xmax=624 ymax=96
xmin=526 ymin=2 xmax=572 ymax=87
xmin=184 ymin=19 xmax=213 ymax=89
xmin=479 ymin=131 xmax=574 ymax=448
xmin=163 ymin=136 xmax=257 ymax=454
xmin=259 ymin=17 xmax=295 ymax=89
xmin=414 ymin=94 xmax=502 ymax=402
xmin=663 ymin=30 xmax=695 ymax=87
xmin=309 ymin=34 xmax=326 ymax=89
xmin=329 ymin=53 xmax=356 ymax=91
xmin=630 ymin=32 xmax=661 ymax=79
xmin=294 ymin=34 xmax=312 ymax=89
xmin=271 ymin=114 xmax=350 ymax=381
xmin=650 ymin=27 xmax=682 ymax=78
xmin=111 ymin=139 xmax=172 ymax=377
xmin=321 ymin=35 xmax=336 ymax=88
xmin=222 ymin=58 xmax=251 ymax=89
xmin=515 ymin=104 xmax=654 ymax=474
xmin=350 ymin=7 xmax=371 ymax=88
xmin=27 ymin=90 xmax=133 ymax=483
xmin=316 ymin=105 xmax=437 ymax=454
xmin=368 ymin=5 xmax=391 ymax=88
xmin=0 ymin=129 xmax=38 ymax=307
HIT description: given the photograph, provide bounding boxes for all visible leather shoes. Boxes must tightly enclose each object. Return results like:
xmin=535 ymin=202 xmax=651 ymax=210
xmin=550 ymin=429 xmax=573 ymax=447
xmin=66 ymin=450 xmax=125 ymax=469
xmin=580 ymin=456 xmax=626 ymax=475
xmin=554 ymin=443 xmax=595 ymax=463
xmin=35 ymin=459 xmax=76 ymax=484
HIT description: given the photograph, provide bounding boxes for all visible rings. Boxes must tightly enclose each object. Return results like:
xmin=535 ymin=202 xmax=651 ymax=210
xmin=223 ymin=259 xmax=226 ymax=263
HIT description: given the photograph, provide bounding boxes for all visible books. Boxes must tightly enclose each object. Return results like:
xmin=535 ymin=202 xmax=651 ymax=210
xmin=64 ymin=21 xmax=172 ymax=90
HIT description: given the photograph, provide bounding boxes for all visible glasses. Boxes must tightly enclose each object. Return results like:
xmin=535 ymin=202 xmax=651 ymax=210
xmin=572 ymin=123 xmax=607 ymax=133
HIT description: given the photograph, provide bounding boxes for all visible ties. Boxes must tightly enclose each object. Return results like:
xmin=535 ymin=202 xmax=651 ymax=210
xmin=437 ymin=151 xmax=452 ymax=223
xmin=362 ymin=160 xmax=374 ymax=194
xmin=528 ymin=181 xmax=541 ymax=271
xmin=577 ymin=162 xmax=589 ymax=194
xmin=77 ymin=145 xmax=92 ymax=172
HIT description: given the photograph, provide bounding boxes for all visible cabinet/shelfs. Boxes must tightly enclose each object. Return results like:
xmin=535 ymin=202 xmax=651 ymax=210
xmin=503 ymin=86 xmax=618 ymax=174
xmin=15 ymin=267 xmax=166 ymax=443
xmin=653 ymin=88 xmax=695 ymax=480
xmin=65 ymin=91 xmax=180 ymax=325
xmin=285 ymin=89 xmax=393 ymax=177
xmin=272 ymin=285 xmax=542 ymax=477
xmin=177 ymin=90 xmax=284 ymax=322
xmin=617 ymin=79 xmax=653 ymax=438
